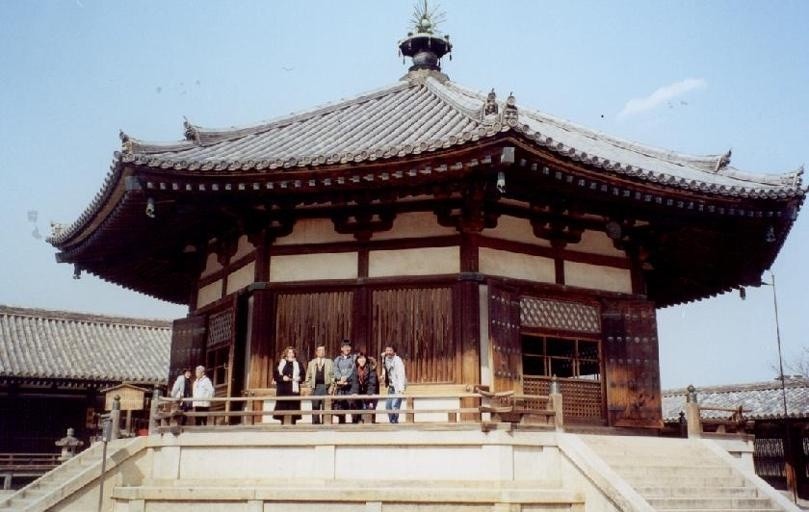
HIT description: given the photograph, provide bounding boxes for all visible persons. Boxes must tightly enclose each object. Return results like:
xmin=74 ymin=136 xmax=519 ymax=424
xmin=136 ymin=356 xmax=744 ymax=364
xmin=304 ymin=344 xmax=335 ymax=424
xmin=272 ymin=344 xmax=305 ymax=424
xmin=333 ymin=337 xmax=359 ymax=424
xmin=379 ymin=342 xmax=407 ymax=424
xmin=190 ymin=364 xmax=216 ymax=424
xmin=169 ymin=367 xmax=193 ymax=425
xmin=355 ymin=350 xmax=381 ymax=424
